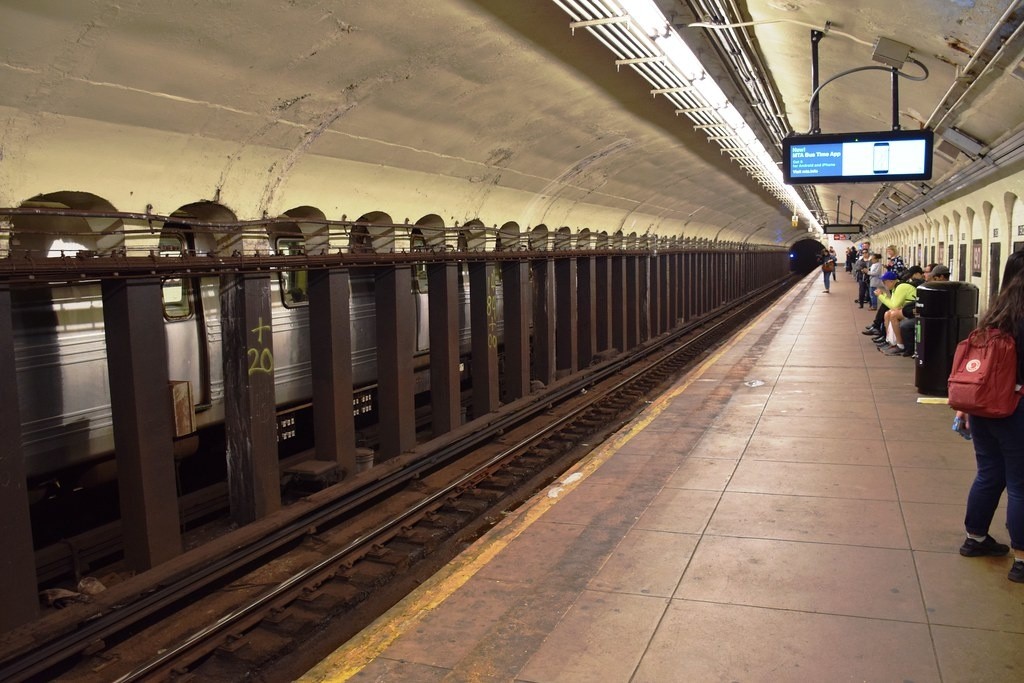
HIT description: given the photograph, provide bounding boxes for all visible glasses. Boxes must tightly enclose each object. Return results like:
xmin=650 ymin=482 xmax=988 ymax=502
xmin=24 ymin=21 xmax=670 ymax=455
xmin=862 ymin=252 xmax=867 ymax=254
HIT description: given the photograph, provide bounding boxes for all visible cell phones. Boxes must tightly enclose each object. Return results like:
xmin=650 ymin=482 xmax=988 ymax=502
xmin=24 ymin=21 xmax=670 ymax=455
xmin=873 ymin=143 xmax=890 ymax=173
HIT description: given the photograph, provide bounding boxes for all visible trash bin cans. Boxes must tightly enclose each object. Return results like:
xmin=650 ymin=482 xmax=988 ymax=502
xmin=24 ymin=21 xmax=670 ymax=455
xmin=914 ymin=281 xmax=980 ymax=397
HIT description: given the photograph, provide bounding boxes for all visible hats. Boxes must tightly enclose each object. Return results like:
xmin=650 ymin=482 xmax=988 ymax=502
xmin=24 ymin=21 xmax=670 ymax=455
xmin=879 ymin=272 xmax=896 ymax=280
xmin=910 ymin=266 xmax=925 ymax=274
xmin=929 ymin=265 xmax=948 ymax=276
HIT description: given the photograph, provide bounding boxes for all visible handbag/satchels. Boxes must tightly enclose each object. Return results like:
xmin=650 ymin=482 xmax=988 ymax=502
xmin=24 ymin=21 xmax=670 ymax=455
xmin=822 ymin=262 xmax=834 ymax=272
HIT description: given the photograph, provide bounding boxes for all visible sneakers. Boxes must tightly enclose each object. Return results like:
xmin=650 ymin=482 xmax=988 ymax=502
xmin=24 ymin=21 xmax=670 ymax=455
xmin=1007 ymin=558 xmax=1024 ymax=583
xmin=959 ymin=533 xmax=1010 ymax=558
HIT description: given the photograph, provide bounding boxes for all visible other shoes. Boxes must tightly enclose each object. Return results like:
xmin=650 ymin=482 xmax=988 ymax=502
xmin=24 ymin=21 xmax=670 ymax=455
xmin=865 ymin=326 xmax=873 ymax=329
xmin=823 ymin=290 xmax=829 ymax=293
xmin=868 ymin=307 xmax=877 ymax=311
xmin=861 ymin=327 xmax=881 ymax=335
xmin=872 ymin=335 xmax=906 ymax=356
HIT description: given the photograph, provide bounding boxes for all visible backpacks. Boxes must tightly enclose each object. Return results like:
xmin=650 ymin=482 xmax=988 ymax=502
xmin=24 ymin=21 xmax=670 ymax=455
xmin=947 ymin=326 xmax=1024 ymax=418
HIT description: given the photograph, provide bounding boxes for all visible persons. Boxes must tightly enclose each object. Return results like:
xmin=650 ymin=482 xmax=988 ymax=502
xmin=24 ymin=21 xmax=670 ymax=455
xmin=845 ymin=240 xmax=952 ymax=358
xmin=954 ymin=248 xmax=1024 ymax=584
xmin=819 ymin=245 xmax=837 ymax=293
